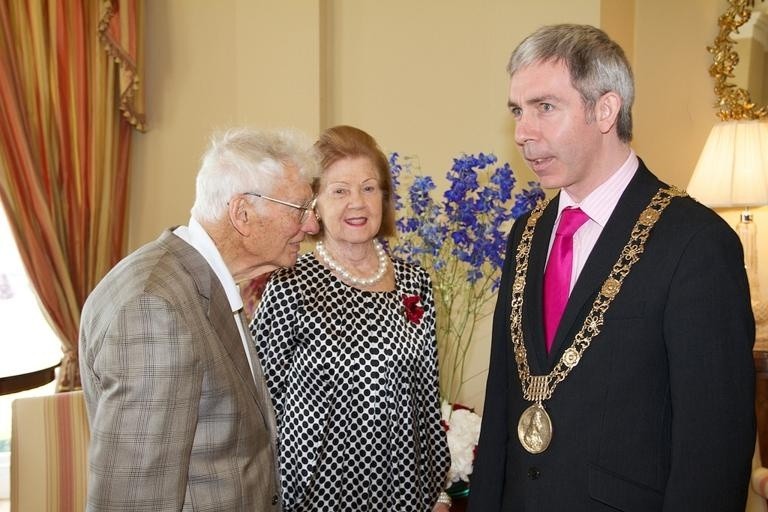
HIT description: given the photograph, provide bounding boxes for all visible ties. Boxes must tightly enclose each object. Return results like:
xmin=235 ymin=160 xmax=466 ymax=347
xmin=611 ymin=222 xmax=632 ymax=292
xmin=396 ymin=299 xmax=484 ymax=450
xmin=543 ymin=205 xmax=589 ymax=352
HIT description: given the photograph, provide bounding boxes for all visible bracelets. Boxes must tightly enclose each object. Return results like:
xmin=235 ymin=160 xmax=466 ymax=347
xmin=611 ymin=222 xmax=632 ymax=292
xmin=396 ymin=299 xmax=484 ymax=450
xmin=436 ymin=491 xmax=453 ymax=508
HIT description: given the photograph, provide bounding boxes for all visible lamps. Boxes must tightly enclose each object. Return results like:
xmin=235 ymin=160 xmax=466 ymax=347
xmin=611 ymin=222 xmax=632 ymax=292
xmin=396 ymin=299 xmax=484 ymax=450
xmin=687 ymin=119 xmax=768 ymax=341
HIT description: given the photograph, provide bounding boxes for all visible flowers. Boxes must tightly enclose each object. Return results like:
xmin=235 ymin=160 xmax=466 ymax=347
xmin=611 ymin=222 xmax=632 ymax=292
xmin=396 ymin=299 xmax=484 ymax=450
xmin=385 ymin=151 xmax=545 ymax=426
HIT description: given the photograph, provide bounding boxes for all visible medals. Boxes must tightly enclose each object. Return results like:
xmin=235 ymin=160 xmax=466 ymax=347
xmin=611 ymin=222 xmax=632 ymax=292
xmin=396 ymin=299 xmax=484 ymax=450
xmin=517 ymin=403 xmax=552 ymax=454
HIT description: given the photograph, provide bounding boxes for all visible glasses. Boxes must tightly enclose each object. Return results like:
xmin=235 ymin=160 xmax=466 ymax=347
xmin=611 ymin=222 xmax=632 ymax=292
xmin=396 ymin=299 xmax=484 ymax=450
xmin=226 ymin=192 xmax=320 ymax=225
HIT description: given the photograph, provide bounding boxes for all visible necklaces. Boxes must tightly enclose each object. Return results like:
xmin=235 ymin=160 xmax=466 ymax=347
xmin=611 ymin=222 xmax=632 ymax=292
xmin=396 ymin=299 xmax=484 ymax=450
xmin=313 ymin=238 xmax=387 ymax=286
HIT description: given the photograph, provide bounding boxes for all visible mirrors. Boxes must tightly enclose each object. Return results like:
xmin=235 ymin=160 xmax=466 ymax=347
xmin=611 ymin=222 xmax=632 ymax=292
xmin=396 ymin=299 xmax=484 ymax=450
xmin=706 ymin=0 xmax=768 ymax=122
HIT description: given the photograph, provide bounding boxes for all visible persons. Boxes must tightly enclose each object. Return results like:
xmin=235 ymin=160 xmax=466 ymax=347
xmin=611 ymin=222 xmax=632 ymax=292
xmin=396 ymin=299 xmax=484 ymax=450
xmin=463 ymin=23 xmax=758 ymax=511
xmin=78 ymin=128 xmax=320 ymax=511
xmin=243 ymin=123 xmax=456 ymax=512
